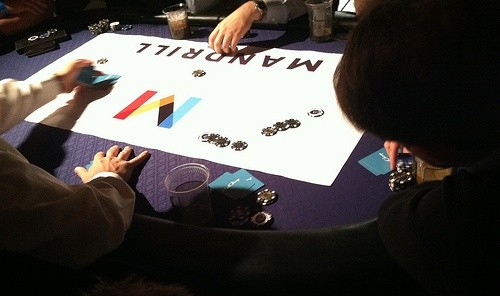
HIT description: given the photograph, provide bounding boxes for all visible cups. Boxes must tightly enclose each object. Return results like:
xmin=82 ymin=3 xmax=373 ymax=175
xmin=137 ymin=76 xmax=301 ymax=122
xmin=162 ymin=3 xmax=190 ymax=39
xmin=164 ymin=163 xmax=214 ymax=220
xmin=305 ymin=0 xmax=334 ymax=41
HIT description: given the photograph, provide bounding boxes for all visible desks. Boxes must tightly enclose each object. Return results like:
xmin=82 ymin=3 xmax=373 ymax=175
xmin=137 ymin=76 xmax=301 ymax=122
xmin=1 ymin=6 xmax=411 ymax=239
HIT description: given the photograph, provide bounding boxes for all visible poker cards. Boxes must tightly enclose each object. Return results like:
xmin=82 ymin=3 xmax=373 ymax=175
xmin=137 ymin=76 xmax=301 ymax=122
xmin=208 ymin=167 xmax=264 ymax=221
xmin=78 ymin=72 xmax=121 ymax=90
xmin=358 ymin=147 xmax=394 ymax=177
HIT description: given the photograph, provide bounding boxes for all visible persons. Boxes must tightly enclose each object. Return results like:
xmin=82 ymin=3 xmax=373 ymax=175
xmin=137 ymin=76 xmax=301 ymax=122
xmin=1 ymin=59 xmax=151 ymax=296
xmin=333 ymin=2 xmax=499 ymax=296
xmin=1 ymin=0 xmax=58 ymax=42
xmin=186 ymin=0 xmax=342 ymax=57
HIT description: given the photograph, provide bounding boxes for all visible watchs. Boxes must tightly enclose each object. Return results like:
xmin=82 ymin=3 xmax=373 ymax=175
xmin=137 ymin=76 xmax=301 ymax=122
xmin=252 ymin=1 xmax=268 ymax=23
